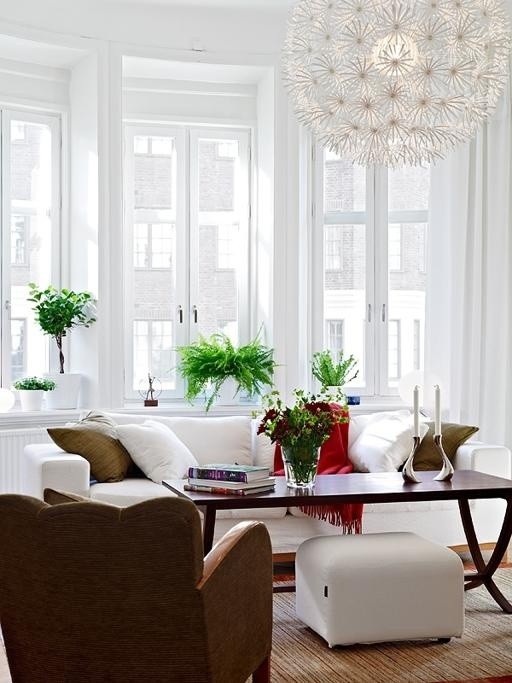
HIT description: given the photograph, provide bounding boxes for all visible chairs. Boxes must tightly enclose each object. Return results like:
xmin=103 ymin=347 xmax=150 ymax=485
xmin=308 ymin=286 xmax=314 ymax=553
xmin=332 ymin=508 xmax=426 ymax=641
xmin=0 ymin=492 xmax=274 ymax=681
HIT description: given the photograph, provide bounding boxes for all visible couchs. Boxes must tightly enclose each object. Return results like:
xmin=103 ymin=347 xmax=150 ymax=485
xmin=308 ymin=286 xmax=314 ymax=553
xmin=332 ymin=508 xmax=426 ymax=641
xmin=19 ymin=413 xmax=512 ymax=567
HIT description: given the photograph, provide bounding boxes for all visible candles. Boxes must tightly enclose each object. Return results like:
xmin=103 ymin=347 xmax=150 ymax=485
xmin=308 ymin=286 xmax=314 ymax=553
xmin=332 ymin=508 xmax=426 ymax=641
xmin=413 ymin=383 xmax=421 ymax=435
xmin=434 ymin=383 xmax=441 ymax=434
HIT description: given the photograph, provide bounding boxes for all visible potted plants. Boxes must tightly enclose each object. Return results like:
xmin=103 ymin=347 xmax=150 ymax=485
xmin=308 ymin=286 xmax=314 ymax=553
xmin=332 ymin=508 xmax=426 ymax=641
xmin=22 ymin=284 xmax=100 ymax=410
xmin=303 ymin=345 xmax=361 ymax=408
xmin=13 ymin=376 xmax=54 ymax=412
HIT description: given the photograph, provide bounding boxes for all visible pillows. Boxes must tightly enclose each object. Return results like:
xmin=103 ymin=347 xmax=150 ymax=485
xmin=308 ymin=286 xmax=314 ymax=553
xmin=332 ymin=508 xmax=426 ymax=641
xmin=112 ymin=418 xmax=199 ymax=483
xmin=47 ymin=410 xmax=130 ymax=483
xmin=347 ymin=411 xmax=430 ymax=475
xmin=394 ymin=419 xmax=483 ymax=476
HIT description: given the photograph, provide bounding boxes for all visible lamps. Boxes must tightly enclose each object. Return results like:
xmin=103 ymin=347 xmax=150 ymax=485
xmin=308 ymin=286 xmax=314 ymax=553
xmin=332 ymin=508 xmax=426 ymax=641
xmin=283 ymin=2 xmax=511 ymax=174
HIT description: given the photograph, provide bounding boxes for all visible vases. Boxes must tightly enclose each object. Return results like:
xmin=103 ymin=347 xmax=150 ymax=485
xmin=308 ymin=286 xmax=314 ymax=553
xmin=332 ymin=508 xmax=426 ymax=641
xmin=276 ymin=438 xmax=327 ymax=490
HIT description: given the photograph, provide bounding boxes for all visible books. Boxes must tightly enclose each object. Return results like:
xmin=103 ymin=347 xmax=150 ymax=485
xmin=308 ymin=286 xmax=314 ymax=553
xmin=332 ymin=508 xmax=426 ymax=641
xmin=189 ymin=478 xmax=276 ymax=488
xmin=184 ymin=483 xmax=275 ymax=496
xmin=188 ymin=462 xmax=270 ymax=482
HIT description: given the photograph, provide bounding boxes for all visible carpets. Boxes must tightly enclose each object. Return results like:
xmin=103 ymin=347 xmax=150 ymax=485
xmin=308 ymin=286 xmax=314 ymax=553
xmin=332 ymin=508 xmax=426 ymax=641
xmin=248 ymin=565 xmax=512 ymax=683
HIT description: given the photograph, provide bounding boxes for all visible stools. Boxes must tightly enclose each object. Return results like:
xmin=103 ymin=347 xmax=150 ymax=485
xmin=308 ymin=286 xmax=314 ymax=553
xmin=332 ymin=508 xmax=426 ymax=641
xmin=292 ymin=530 xmax=467 ymax=647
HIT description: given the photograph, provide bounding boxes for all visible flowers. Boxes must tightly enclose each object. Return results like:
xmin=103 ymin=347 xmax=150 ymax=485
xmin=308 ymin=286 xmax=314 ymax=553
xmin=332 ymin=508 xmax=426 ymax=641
xmin=250 ymin=384 xmax=353 ymax=447
